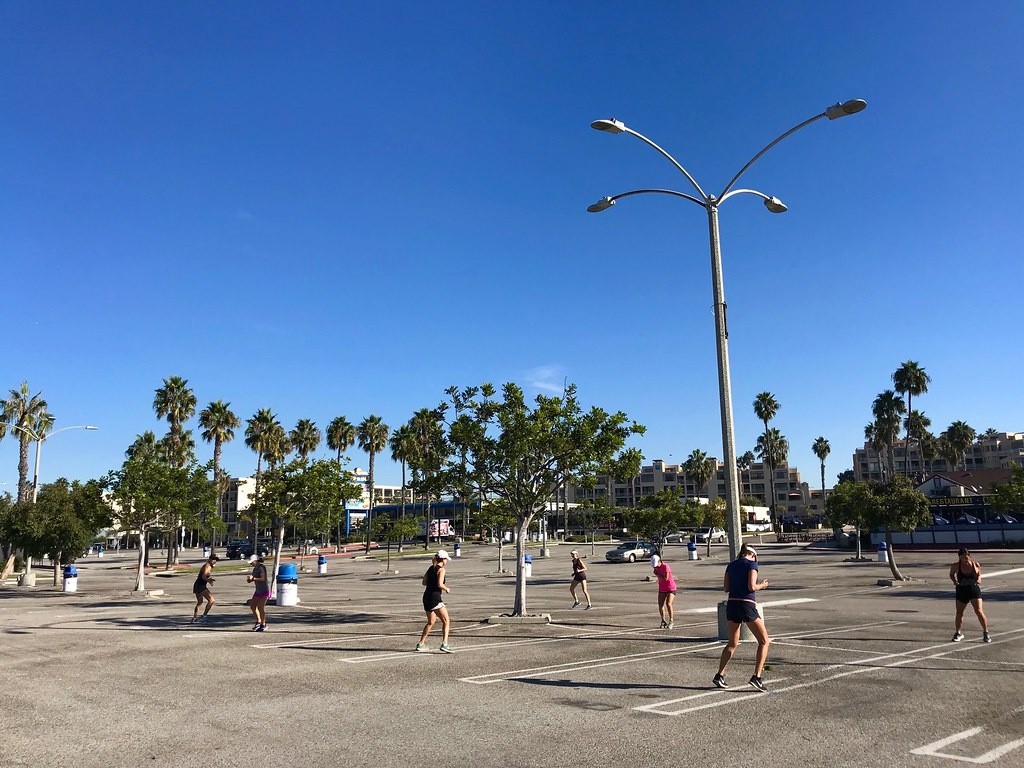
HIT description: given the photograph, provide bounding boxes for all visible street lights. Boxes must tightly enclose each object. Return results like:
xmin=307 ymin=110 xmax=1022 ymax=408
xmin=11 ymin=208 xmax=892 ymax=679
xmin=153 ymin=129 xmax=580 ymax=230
xmin=0 ymin=422 xmax=98 ymax=586
xmin=587 ymin=99 xmax=867 ymax=639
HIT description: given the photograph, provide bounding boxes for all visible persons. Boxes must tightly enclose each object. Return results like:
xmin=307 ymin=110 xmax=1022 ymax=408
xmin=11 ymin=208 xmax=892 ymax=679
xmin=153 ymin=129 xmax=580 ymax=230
xmin=246 ymin=554 xmax=271 ymax=632
xmin=651 ymin=555 xmax=677 ymax=629
xmin=712 ymin=543 xmax=770 ymax=693
xmin=570 ymin=551 xmax=593 ymax=610
xmin=185 ymin=539 xmax=189 ymax=548
xmin=191 ymin=554 xmax=220 ymax=625
xmin=416 ymin=550 xmax=455 ymax=653
xmin=950 ymin=547 xmax=992 ymax=643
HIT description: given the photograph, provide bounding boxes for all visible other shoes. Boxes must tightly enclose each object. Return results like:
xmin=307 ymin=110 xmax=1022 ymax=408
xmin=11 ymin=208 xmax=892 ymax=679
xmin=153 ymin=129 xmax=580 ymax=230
xmin=191 ymin=618 xmax=201 ymax=623
xmin=200 ymin=616 xmax=208 ymax=624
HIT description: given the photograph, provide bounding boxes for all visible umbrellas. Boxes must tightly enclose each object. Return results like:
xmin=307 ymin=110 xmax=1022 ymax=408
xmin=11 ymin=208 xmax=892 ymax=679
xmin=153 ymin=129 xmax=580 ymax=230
xmin=927 ymin=512 xmax=1018 ymax=525
xmin=784 ymin=515 xmax=825 ymax=533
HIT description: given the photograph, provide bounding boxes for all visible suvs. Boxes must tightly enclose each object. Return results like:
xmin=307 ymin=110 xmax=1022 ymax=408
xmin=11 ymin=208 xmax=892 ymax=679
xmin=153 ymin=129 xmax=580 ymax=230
xmin=226 ymin=539 xmax=269 ymax=559
xmin=690 ymin=526 xmax=726 ymax=543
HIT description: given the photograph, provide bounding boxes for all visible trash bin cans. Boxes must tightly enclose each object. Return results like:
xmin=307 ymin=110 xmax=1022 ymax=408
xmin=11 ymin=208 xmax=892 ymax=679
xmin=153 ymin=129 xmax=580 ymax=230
xmin=525 ymin=554 xmax=531 ymax=577
xmin=63 ymin=566 xmax=78 ymax=592
xmin=318 ymin=556 xmax=328 ymax=576
xmin=98 ymin=549 xmax=103 ymax=558
xmin=454 ymin=544 xmax=461 ymax=557
xmin=687 ymin=542 xmax=698 ymax=560
xmin=276 ymin=563 xmax=298 ymax=606
xmin=203 ymin=547 xmax=209 ymax=558
xmin=877 ymin=542 xmax=889 ymax=562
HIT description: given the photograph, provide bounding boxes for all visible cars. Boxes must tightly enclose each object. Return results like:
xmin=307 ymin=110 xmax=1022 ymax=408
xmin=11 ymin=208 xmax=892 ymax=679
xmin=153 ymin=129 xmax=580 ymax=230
xmin=786 ymin=517 xmax=804 ymax=525
xmin=91 ymin=542 xmax=101 ymax=550
xmin=811 ymin=516 xmax=825 ymax=524
xmin=605 ymin=541 xmax=659 ymax=563
xmin=931 ymin=513 xmax=1018 ymax=524
xmin=651 ymin=530 xmax=685 ymax=543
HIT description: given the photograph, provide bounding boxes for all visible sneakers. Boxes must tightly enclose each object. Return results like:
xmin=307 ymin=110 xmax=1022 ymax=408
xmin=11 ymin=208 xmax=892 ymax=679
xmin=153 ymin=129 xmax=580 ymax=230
xmin=416 ymin=644 xmax=430 ymax=651
xmin=257 ymin=624 xmax=268 ymax=632
xmin=983 ymin=631 xmax=991 ymax=643
xmin=252 ymin=623 xmax=261 ymax=631
xmin=660 ymin=622 xmax=668 ymax=628
xmin=713 ymin=674 xmax=728 ymax=688
xmin=584 ymin=605 xmax=592 ymax=610
xmin=440 ymin=642 xmax=455 ymax=653
xmin=571 ymin=601 xmax=581 ymax=608
xmin=748 ymin=675 xmax=766 ymax=692
xmin=667 ymin=621 xmax=674 ymax=628
xmin=952 ymin=633 xmax=965 ymax=642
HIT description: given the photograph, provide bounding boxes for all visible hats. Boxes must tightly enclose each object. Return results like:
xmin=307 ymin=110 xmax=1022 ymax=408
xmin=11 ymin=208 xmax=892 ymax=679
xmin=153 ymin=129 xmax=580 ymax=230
xmin=570 ymin=551 xmax=577 ymax=554
xmin=746 ymin=546 xmax=758 ymax=563
xmin=437 ymin=550 xmax=451 ymax=561
xmin=651 ymin=555 xmax=660 ymax=568
xmin=209 ymin=554 xmax=219 ymax=561
xmin=247 ymin=554 xmax=260 ymax=563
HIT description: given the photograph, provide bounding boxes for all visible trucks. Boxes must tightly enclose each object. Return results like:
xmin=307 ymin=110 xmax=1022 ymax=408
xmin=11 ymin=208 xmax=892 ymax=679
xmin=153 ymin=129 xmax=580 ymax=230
xmin=417 ymin=519 xmax=455 ymax=542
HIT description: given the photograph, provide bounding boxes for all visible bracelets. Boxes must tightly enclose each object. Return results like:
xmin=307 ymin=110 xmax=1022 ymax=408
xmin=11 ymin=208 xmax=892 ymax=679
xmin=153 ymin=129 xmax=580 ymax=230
xmin=252 ymin=577 xmax=255 ymax=581
